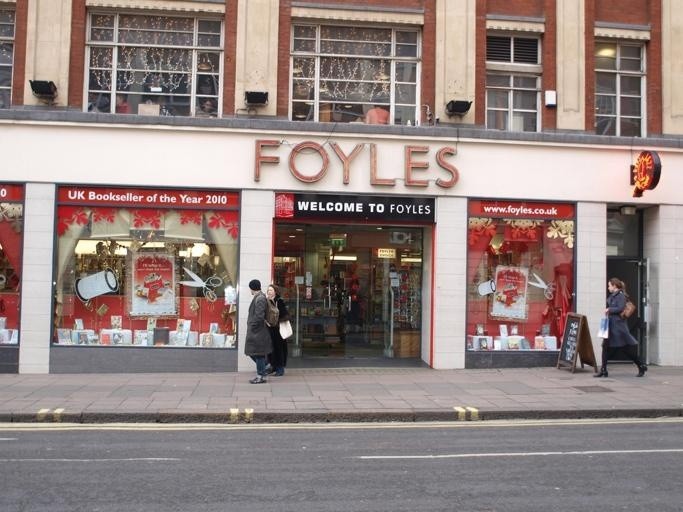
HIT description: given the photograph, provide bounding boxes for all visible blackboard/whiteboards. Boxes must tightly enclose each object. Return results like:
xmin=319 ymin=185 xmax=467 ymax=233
xmin=558 ymin=312 xmax=584 ymax=368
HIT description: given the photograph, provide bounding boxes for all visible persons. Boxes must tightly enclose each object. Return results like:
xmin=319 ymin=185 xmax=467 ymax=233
xmin=593 ymin=277 xmax=648 ymax=378
xmin=320 ymin=280 xmax=329 ymax=299
xmin=243 ymin=279 xmax=274 ymax=384
xmin=91 ymin=91 xmax=391 ymax=124
xmin=265 ymin=284 xmax=289 ymax=376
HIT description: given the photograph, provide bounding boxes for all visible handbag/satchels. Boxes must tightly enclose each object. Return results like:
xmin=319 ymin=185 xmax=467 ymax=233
xmin=279 ymin=320 xmax=293 ymax=340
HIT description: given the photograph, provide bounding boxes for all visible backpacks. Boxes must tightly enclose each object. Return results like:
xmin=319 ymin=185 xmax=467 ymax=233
xmin=254 ymin=293 xmax=279 ymax=327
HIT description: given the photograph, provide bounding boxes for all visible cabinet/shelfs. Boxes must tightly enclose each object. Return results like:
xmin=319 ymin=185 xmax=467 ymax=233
xmin=281 ymin=299 xmax=342 ymax=344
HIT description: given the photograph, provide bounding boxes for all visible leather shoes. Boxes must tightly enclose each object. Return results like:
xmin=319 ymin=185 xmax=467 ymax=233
xmin=249 ymin=376 xmax=266 ymax=384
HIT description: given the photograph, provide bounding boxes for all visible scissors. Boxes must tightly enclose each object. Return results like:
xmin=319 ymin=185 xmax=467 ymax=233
xmin=527 ymin=273 xmax=557 ymax=300
xmin=178 ymin=267 xmax=223 ymax=302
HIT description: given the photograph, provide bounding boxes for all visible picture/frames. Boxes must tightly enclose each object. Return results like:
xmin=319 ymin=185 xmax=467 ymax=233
xmin=123 ymin=247 xmax=180 ymax=321
xmin=488 ymin=262 xmax=533 ymax=324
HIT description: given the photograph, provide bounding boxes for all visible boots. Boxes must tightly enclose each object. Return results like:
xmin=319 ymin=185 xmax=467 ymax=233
xmin=637 ymin=365 xmax=648 ymax=378
xmin=593 ymin=367 xmax=608 ymax=377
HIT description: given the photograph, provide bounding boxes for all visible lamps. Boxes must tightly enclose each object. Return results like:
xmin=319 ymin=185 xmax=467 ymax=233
xmin=445 ymin=100 xmax=472 ymax=118
xmin=29 ymin=80 xmax=58 ymax=99
xmin=244 ymin=91 xmax=268 ymax=107
